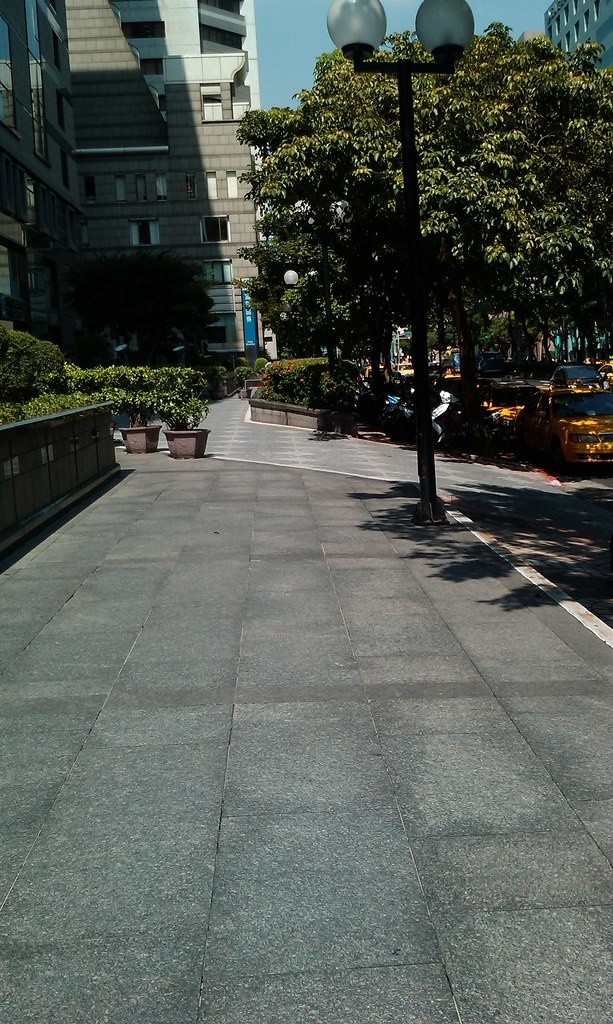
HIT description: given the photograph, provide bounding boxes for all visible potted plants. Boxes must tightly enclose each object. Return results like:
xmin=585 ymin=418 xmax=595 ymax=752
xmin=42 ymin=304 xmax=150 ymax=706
xmin=117 ymin=364 xmax=162 ymax=453
xmin=234 ymin=367 xmax=254 ymax=399
xmin=155 ymin=366 xmax=212 ymax=459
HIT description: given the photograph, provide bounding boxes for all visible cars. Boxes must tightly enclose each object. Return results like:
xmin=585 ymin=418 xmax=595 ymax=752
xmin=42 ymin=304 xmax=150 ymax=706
xmin=514 ymin=387 xmax=613 ymax=471
xmin=448 ymin=353 xmax=461 ymax=374
xmin=599 ymin=362 xmax=613 ymax=385
xmin=550 ymin=364 xmax=610 ymax=392
xmin=393 ymin=361 xmax=540 ymax=445
xmin=479 ymin=350 xmax=505 ymax=375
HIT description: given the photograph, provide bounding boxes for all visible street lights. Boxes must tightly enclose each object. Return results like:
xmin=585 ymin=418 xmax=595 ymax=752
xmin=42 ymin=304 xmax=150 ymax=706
xmin=284 ymin=269 xmax=322 ymax=336
xmin=324 ymin=0 xmax=473 ymax=525
